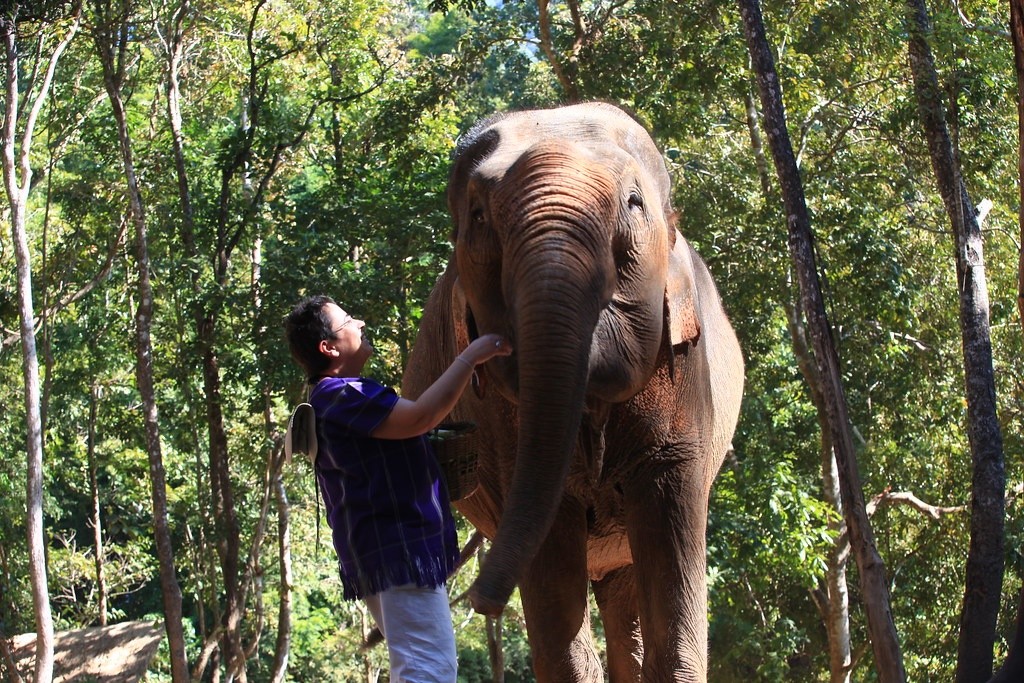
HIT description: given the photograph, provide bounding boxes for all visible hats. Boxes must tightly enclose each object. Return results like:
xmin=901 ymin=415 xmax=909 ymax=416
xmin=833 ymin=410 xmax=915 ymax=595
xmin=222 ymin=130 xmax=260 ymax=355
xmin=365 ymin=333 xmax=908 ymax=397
xmin=285 ymin=403 xmax=319 ymax=469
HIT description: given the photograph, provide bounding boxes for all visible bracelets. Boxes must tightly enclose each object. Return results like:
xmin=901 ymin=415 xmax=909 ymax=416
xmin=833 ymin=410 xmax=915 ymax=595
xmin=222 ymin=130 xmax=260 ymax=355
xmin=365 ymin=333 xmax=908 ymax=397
xmin=456 ymin=357 xmax=478 ymax=386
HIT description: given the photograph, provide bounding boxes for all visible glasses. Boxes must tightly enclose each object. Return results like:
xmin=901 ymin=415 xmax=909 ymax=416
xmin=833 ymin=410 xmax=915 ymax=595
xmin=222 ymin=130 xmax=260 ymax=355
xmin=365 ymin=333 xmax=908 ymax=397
xmin=325 ymin=314 xmax=356 ymax=339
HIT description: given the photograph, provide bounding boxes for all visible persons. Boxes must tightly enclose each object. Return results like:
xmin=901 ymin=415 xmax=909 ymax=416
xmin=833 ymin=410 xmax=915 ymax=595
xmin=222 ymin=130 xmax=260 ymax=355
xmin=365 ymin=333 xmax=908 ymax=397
xmin=284 ymin=295 xmax=512 ymax=683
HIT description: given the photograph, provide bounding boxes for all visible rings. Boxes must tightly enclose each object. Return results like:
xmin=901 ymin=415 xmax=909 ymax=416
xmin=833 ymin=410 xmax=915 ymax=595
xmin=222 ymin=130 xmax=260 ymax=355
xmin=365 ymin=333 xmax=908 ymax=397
xmin=496 ymin=341 xmax=500 ymax=347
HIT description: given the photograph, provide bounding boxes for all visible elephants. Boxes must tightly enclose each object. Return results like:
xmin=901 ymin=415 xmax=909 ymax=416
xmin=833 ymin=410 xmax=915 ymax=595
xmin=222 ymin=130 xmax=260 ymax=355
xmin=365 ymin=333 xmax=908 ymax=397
xmin=391 ymin=95 xmax=749 ymax=683
